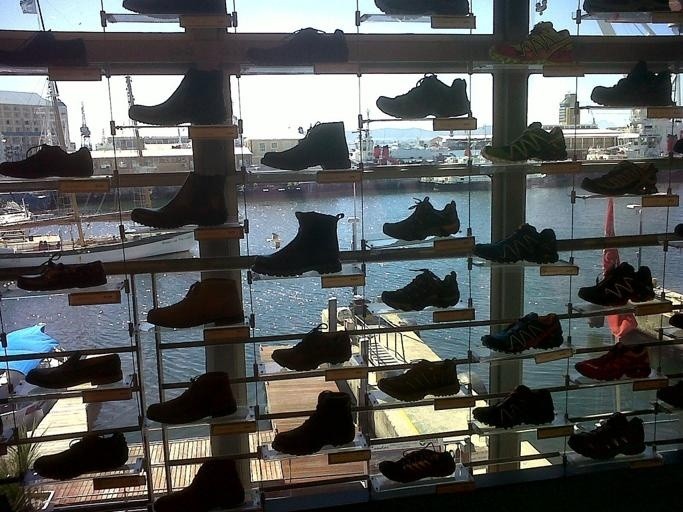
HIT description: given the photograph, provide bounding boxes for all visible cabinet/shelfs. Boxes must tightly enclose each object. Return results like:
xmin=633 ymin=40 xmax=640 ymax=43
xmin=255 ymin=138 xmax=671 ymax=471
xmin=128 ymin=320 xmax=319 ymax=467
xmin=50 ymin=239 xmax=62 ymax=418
xmin=0 ymin=0 xmax=683 ymax=512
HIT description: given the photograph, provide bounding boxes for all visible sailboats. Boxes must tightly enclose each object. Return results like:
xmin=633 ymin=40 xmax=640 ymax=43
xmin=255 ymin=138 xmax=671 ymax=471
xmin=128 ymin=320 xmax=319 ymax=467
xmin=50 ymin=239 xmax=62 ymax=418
xmin=349 ymin=109 xmax=448 ymax=163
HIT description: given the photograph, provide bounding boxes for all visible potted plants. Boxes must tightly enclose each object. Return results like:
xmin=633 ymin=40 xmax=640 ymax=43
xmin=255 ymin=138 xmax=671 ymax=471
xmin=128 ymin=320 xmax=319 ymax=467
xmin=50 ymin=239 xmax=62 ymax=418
xmin=0 ymin=415 xmax=56 ymax=512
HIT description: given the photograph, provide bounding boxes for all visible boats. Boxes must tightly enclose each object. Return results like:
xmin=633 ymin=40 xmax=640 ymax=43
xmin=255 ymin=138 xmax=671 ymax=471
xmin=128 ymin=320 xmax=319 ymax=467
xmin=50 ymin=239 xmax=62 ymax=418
xmin=573 ymin=280 xmax=681 ymax=377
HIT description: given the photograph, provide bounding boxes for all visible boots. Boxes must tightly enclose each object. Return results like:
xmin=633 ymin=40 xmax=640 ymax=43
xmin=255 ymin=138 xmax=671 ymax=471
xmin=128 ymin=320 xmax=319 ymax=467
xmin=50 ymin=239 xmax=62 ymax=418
xmin=260 ymin=122 xmax=351 ymax=171
xmin=252 ymin=210 xmax=345 ymax=278
xmin=147 ymin=278 xmax=244 ymax=328
xmin=154 ymin=460 xmax=244 ymax=512
xmin=131 ymin=171 xmax=228 ymax=229
xmin=273 ymin=391 xmax=355 ymax=455
xmin=128 ymin=70 xmax=227 ymax=124
xmin=146 ymin=373 xmax=237 ymax=424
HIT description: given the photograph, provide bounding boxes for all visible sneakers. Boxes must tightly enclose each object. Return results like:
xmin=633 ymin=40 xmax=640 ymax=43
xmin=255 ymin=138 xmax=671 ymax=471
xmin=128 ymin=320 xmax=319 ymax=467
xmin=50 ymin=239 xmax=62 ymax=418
xmin=473 ymin=224 xmax=559 ymax=264
xmin=382 ymin=269 xmax=460 ymax=311
xmin=575 ymin=342 xmax=650 ymax=382
xmin=272 ymin=330 xmax=351 ymax=372
xmin=591 ymin=62 xmax=671 ymax=107
xmin=383 ymin=199 xmax=460 ymax=241
xmin=481 ymin=312 xmax=563 ymax=354
xmin=580 ymin=160 xmax=659 ymax=195
xmin=0 ymin=145 xmax=94 ymax=178
xmin=375 ymin=0 xmax=469 ymax=21
xmin=659 ymin=382 xmax=683 ymax=410
xmin=378 ymin=359 xmax=459 ymax=402
xmin=669 ymin=314 xmax=682 ymax=329
xmin=481 ymin=122 xmax=568 ymax=163
xmin=567 ymin=412 xmax=645 ymax=460
xmin=584 ymin=0 xmax=672 ymax=23
xmin=376 ymin=76 xmax=470 ymax=119
xmin=488 ymin=21 xmax=576 ymax=65
xmin=472 ymin=385 xmax=554 ymax=429
xmin=0 ymin=30 xmax=89 ymax=66
xmin=577 ymin=262 xmax=655 ymax=307
xmin=379 ymin=450 xmax=455 ymax=483
xmin=246 ymin=28 xmax=349 ymax=65
xmin=123 ymin=0 xmax=226 ymax=19
xmin=33 ymin=431 xmax=128 ymax=480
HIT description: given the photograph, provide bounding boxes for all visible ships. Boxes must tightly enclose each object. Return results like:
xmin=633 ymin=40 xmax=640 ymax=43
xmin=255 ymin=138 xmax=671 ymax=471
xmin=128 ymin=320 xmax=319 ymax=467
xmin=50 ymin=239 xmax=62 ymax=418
xmin=440 ymin=101 xmax=683 ymax=160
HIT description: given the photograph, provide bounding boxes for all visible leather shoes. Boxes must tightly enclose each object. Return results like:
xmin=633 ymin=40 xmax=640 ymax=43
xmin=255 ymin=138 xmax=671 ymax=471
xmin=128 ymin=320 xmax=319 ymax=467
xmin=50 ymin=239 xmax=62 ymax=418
xmin=18 ymin=260 xmax=107 ymax=291
xmin=25 ymin=352 xmax=123 ymax=389
